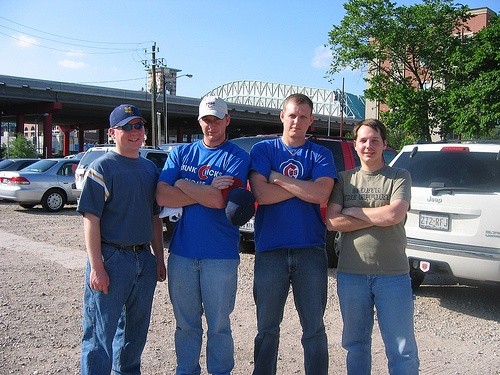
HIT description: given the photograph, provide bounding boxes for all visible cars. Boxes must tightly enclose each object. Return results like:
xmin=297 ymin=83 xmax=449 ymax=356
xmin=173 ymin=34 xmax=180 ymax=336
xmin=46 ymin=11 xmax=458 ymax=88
xmin=73 ymin=146 xmax=172 ymax=206
xmin=0 ymin=159 xmax=40 ymax=172
xmin=232 ymin=134 xmax=401 ymax=269
xmin=0 ymin=158 xmax=80 ymax=213
xmin=388 ymin=140 xmax=500 ymax=294
xmin=63 ymin=152 xmax=87 ymax=174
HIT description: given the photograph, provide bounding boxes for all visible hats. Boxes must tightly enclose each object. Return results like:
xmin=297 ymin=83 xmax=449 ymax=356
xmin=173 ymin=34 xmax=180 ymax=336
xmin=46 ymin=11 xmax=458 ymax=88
xmin=110 ymin=104 xmax=147 ymax=128
xmin=198 ymin=95 xmax=228 ymax=121
xmin=221 ymin=178 xmax=258 ymax=227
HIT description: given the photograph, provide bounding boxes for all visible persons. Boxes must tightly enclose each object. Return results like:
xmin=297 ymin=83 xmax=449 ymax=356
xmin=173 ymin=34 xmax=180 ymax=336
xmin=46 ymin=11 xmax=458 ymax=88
xmin=77 ymin=105 xmax=166 ymax=375
xmin=155 ymin=94 xmax=249 ymax=375
xmin=325 ymin=118 xmax=420 ymax=375
xmin=251 ymin=93 xmax=337 ymax=375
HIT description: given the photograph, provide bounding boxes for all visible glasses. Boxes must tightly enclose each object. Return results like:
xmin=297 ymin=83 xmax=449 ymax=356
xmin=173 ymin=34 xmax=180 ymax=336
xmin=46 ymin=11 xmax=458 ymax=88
xmin=116 ymin=121 xmax=144 ymax=131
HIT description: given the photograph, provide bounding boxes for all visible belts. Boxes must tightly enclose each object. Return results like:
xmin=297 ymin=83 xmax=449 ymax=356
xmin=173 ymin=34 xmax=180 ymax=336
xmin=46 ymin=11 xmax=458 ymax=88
xmin=101 ymin=237 xmax=150 ymax=252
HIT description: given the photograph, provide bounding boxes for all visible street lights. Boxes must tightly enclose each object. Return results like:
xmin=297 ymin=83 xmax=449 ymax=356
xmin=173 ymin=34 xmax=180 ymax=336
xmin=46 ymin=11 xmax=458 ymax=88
xmin=163 ymin=73 xmax=193 ymax=145
